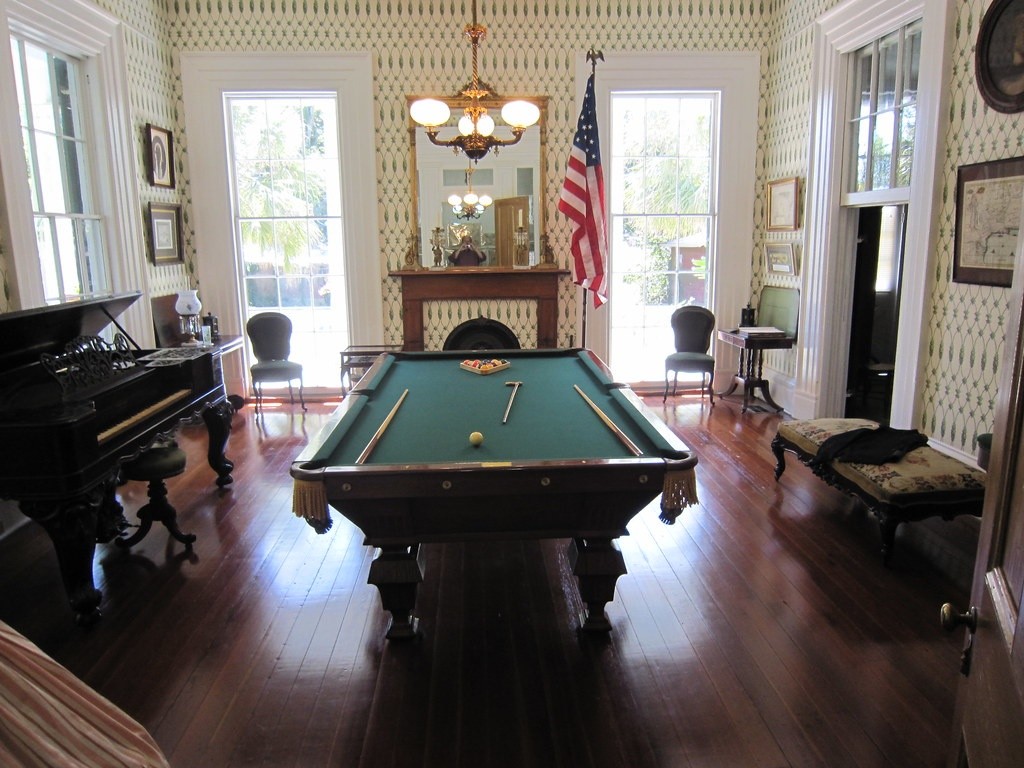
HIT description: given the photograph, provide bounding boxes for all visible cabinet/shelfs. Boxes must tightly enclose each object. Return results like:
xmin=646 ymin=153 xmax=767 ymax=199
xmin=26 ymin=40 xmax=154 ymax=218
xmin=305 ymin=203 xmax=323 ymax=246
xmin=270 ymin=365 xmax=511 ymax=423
xmin=339 ymin=344 xmax=404 ymax=396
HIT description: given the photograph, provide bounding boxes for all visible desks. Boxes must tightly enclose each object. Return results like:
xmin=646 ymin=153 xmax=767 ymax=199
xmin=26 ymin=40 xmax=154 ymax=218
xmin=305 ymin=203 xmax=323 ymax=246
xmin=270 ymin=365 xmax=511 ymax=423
xmin=168 ymin=333 xmax=243 ymax=356
xmin=717 ymin=331 xmax=792 ymax=415
xmin=291 ymin=347 xmax=700 ymax=642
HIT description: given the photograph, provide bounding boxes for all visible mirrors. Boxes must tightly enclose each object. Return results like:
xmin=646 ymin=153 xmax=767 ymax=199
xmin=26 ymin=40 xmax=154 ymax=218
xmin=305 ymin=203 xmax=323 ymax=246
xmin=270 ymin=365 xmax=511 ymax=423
xmin=405 ymin=95 xmax=549 ymax=269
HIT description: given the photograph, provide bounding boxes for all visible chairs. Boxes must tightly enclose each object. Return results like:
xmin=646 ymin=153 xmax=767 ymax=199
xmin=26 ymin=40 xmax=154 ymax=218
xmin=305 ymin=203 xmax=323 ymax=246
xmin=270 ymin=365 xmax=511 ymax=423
xmin=664 ymin=306 xmax=716 ymax=407
xmin=246 ymin=311 xmax=308 ymax=415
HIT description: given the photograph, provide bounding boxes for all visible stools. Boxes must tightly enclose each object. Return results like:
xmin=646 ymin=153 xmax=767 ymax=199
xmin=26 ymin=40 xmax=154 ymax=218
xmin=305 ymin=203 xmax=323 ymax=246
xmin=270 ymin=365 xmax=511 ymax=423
xmin=770 ymin=419 xmax=987 ymax=554
xmin=117 ymin=447 xmax=198 ymax=549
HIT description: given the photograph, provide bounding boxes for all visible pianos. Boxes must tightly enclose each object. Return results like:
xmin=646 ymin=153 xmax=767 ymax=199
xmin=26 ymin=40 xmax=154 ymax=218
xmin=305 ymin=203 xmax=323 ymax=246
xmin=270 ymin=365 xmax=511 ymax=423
xmin=0 ymin=286 xmax=229 ymax=626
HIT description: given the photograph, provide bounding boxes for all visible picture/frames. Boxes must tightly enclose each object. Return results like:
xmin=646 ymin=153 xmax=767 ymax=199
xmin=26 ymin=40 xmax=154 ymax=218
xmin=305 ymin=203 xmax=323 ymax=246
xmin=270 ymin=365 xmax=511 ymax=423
xmin=975 ymin=0 xmax=1024 ymax=114
xmin=146 ymin=123 xmax=176 ymax=191
xmin=148 ymin=201 xmax=186 ymax=267
xmin=951 ymin=155 xmax=1024 ymax=288
xmin=765 ymin=242 xmax=799 ymax=277
xmin=766 ymin=177 xmax=802 ymax=230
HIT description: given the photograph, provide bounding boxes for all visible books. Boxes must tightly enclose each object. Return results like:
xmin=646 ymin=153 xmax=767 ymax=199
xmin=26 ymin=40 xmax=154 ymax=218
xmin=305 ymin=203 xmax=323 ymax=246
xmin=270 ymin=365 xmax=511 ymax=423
xmin=737 ymin=326 xmax=786 ymax=338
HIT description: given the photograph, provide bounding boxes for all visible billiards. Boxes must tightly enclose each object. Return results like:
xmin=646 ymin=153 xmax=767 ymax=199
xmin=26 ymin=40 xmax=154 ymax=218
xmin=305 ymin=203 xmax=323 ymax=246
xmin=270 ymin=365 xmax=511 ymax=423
xmin=464 ymin=359 xmax=507 ymax=369
xmin=469 ymin=432 xmax=483 ymax=445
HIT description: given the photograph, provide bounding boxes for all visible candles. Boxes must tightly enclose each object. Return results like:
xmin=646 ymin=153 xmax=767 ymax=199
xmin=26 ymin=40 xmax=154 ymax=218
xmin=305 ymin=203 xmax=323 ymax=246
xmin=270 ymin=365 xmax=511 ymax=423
xmin=518 ymin=209 xmax=523 ymax=227
xmin=435 ymin=208 xmax=441 ymax=227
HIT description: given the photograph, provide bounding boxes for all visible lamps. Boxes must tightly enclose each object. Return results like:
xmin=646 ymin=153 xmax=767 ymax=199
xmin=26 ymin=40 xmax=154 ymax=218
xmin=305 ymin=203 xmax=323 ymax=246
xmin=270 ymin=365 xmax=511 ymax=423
xmin=175 ymin=288 xmax=202 ymax=346
xmin=409 ymin=0 xmax=540 ymax=164
xmin=448 ymin=159 xmax=493 ymax=219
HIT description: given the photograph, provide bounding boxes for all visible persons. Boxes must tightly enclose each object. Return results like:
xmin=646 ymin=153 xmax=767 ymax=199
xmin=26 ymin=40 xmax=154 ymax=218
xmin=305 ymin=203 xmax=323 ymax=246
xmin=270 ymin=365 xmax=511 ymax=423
xmin=557 ymin=70 xmax=609 ymax=310
xmin=447 ymin=235 xmax=486 ymax=266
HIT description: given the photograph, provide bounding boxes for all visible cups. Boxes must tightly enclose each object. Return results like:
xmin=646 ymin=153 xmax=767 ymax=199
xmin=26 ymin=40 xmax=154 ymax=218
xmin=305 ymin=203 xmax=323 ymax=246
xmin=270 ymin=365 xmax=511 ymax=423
xmin=202 ymin=325 xmax=211 ymax=345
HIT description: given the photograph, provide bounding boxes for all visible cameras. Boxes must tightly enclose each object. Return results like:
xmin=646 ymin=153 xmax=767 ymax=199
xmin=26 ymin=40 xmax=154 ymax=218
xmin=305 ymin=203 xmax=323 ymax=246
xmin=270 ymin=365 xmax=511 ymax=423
xmin=465 ymin=245 xmax=471 ymax=248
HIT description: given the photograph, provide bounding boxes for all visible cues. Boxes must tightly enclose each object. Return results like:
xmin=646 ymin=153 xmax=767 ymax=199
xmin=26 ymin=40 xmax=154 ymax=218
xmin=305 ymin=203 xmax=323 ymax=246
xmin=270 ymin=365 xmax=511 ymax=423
xmin=354 ymin=388 xmax=408 ymax=465
xmin=573 ymin=384 xmax=644 ymax=457
